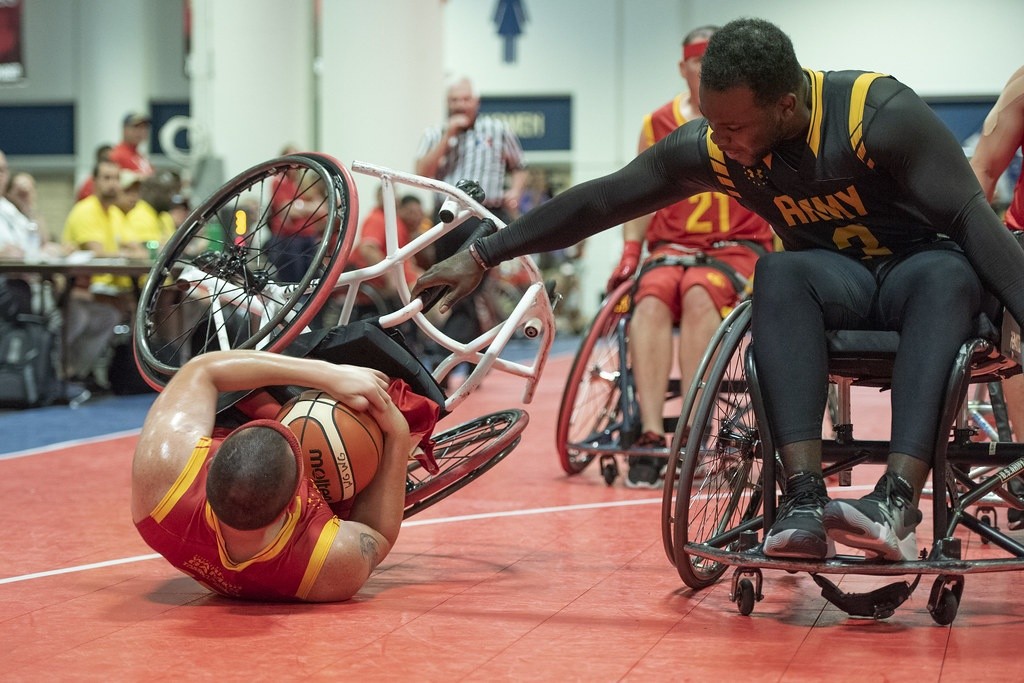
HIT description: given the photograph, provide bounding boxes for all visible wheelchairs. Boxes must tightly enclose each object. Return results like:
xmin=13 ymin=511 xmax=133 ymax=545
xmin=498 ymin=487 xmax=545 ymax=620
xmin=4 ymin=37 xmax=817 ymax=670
xmin=660 ymin=230 xmax=1024 ymax=626
xmin=556 ymin=279 xmax=841 ymax=491
xmin=132 ymin=151 xmax=562 ymax=520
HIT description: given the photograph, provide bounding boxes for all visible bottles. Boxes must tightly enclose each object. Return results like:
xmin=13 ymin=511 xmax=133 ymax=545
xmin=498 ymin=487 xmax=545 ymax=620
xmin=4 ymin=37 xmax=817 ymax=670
xmin=206 ymin=208 xmax=225 ymax=252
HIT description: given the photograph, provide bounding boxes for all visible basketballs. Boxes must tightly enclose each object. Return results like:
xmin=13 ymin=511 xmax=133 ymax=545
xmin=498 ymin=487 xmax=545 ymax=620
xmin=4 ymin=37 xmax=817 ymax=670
xmin=272 ymin=388 xmax=385 ymax=503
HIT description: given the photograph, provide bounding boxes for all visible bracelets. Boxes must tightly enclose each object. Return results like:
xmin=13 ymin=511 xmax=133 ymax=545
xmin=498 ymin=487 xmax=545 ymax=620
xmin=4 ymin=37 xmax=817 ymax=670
xmin=468 ymin=243 xmax=490 ymax=272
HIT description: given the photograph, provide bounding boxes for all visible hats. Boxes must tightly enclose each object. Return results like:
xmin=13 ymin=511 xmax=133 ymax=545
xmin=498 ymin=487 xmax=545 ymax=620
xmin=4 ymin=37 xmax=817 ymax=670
xmin=119 ymin=169 xmax=142 ymax=190
xmin=125 ymin=112 xmax=152 ymax=128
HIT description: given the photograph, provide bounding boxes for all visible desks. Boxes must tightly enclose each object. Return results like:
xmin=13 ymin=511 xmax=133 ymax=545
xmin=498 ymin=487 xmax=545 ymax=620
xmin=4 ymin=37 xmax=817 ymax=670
xmin=1 ymin=258 xmax=153 ymax=404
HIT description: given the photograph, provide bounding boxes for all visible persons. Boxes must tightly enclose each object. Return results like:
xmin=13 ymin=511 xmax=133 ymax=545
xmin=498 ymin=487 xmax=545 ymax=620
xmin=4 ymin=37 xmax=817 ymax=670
xmin=131 ymin=350 xmax=409 ymax=603
xmin=410 ymin=19 xmax=1024 ymax=561
xmin=1 ymin=78 xmax=589 ymax=412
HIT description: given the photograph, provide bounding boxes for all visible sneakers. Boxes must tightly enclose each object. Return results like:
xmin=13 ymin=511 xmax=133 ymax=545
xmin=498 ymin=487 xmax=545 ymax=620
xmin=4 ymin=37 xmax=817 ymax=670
xmin=763 ymin=469 xmax=837 ymax=558
xmin=626 ymin=428 xmax=669 ymax=489
xmin=819 ymin=470 xmax=923 ymax=561
xmin=670 ymin=424 xmax=711 ymax=469
